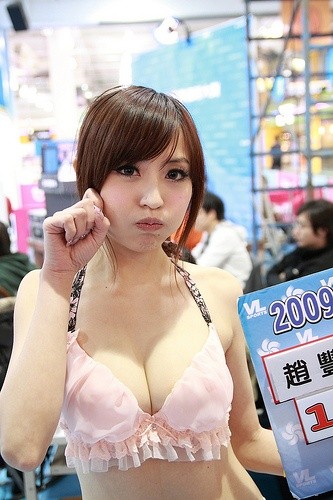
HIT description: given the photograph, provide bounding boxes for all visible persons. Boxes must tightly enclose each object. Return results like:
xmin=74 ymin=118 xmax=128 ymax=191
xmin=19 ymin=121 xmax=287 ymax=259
xmin=0 ymin=222 xmax=41 ymax=500
xmin=0 ymin=86 xmax=287 ymax=500
xmin=255 ymin=199 xmax=333 ymax=435
xmin=191 ymin=192 xmax=253 ymax=288
